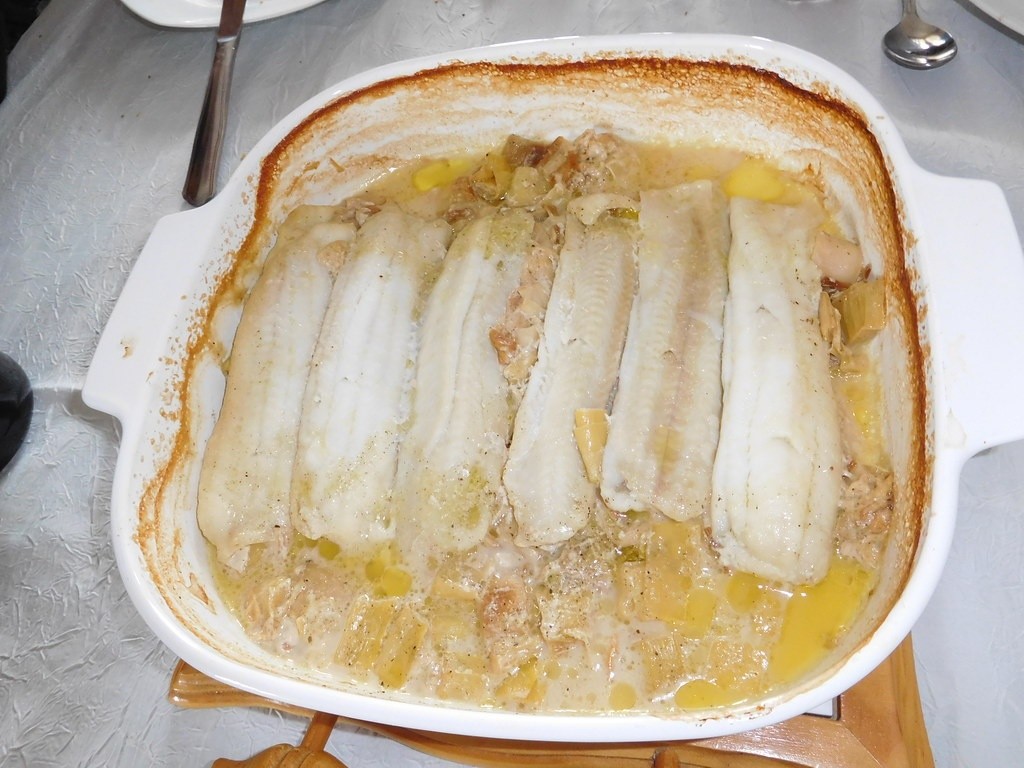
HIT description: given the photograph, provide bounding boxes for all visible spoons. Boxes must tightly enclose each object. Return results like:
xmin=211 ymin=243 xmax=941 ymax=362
xmin=881 ymin=0 xmax=958 ymax=70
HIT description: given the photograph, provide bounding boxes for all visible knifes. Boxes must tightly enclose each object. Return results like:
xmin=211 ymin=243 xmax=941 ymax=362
xmin=181 ymin=0 xmax=247 ymax=208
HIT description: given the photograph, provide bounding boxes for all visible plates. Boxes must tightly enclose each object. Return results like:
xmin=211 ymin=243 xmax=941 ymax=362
xmin=122 ymin=0 xmax=327 ymax=28
xmin=80 ymin=32 xmax=1024 ymax=743
